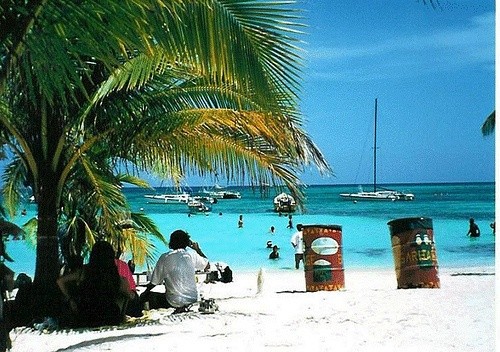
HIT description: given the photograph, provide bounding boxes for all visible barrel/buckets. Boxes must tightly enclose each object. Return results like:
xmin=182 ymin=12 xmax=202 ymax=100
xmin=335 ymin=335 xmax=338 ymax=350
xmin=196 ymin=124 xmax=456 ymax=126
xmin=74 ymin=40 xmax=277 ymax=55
xmin=388 ymin=217 xmax=440 ymax=289
xmin=301 ymin=225 xmax=345 ymax=291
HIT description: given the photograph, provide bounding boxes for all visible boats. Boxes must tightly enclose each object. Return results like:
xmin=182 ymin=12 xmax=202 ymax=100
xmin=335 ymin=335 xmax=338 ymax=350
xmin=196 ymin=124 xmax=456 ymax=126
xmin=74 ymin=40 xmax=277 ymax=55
xmin=144 ymin=192 xmax=191 ymax=203
xmin=187 ymin=196 xmax=212 ymax=212
xmin=274 ymin=192 xmax=297 ymax=212
xmin=203 ymin=188 xmax=241 ymax=199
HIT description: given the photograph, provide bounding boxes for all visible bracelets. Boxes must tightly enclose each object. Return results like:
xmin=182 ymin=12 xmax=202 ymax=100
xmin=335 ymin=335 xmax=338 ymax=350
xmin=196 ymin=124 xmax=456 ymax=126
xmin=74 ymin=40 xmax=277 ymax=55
xmin=67 ymin=297 xmax=72 ymax=302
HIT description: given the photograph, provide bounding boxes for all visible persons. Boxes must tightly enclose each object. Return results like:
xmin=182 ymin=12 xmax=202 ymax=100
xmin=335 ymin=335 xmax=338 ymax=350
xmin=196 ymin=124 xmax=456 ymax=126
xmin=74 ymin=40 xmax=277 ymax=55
xmin=287 ymin=215 xmax=294 ymax=229
xmin=269 ymin=246 xmax=280 ymax=260
xmin=56 ymin=241 xmax=135 ymax=327
xmin=490 ymin=220 xmax=495 ymax=233
xmin=238 ymin=215 xmax=243 ymax=228
xmin=1 ymin=265 xmax=15 ymax=352
xmin=140 ymin=230 xmax=210 ymax=308
xmin=291 ymin=224 xmax=305 ymax=269
xmin=114 ymin=257 xmax=142 ymax=318
xmin=270 ymin=227 xmax=274 ymax=232
xmin=267 ymin=241 xmax=272 ymax=248
xmin=466 ymin=217 xmax=480 ymax=237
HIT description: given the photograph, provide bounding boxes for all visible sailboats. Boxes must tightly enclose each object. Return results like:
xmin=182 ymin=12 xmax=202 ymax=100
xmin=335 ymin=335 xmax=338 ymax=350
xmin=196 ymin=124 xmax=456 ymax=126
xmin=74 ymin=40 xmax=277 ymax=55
xmin=339 ymin=98 xmax=414 ymax=201
